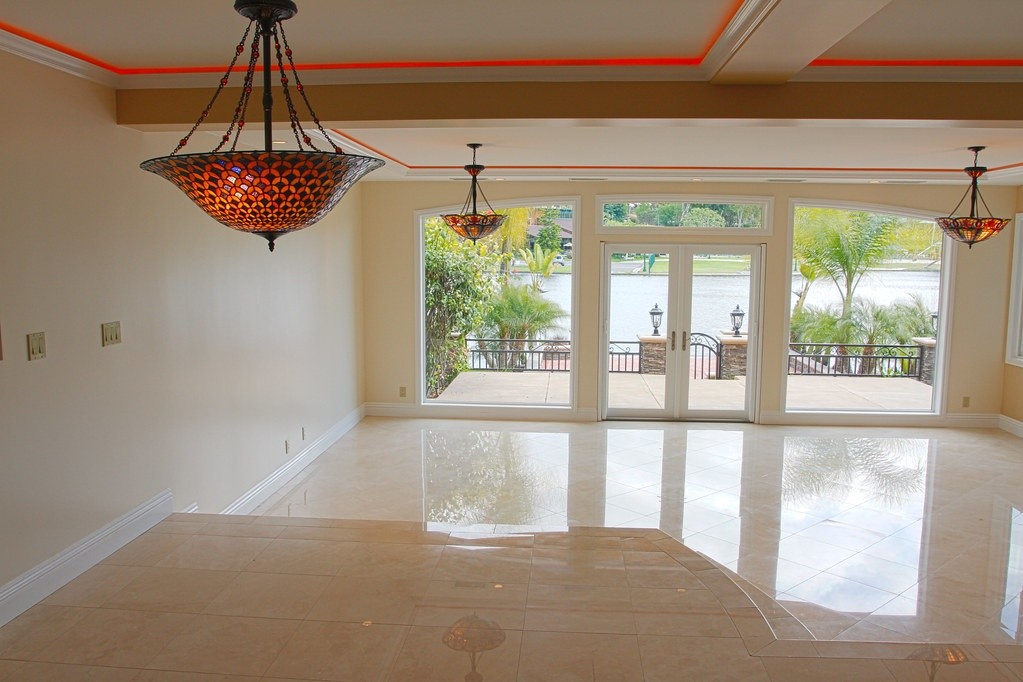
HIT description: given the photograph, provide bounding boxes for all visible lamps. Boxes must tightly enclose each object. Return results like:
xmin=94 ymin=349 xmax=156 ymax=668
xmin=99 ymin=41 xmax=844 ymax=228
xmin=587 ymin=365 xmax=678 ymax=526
xmin=135 ymin=0 xmax=393 ymax=258
xmin=430 ymin=607 xmax=517 ymax=682
xmin=931 ymin=311 xmax=938 ymax=341
xmin=432 ymin=139 xmax=511 ymax=253
xmin=903 ymin=641 xmax=974 ymax=682
xmin=723 ymin=302 xmax=750 ymax=341
xmin=641 ymin=301 xmax=667 ymax=338
xmin=933 ymin=141 xmax=1016 ymax=260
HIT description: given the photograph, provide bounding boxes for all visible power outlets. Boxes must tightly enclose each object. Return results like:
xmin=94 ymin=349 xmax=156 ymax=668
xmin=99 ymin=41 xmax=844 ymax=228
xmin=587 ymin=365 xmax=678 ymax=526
xmin=303 ymin=490 xmax=314 ymax=506
xmin=287 ymin=504 xmax=295 ymax=518
xmin=301 ymin=425 xmax=310 ymax=440
xmin=282 ymin=439 xmax=293 ymax=456
xmin=960 ymin=396 xmax=972 ymax=409
xmin=396 ymin=386 xmax=409 ymax=399
